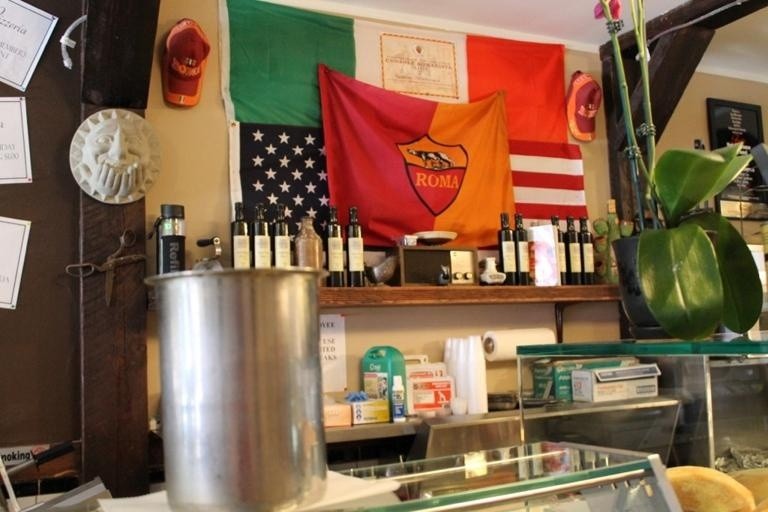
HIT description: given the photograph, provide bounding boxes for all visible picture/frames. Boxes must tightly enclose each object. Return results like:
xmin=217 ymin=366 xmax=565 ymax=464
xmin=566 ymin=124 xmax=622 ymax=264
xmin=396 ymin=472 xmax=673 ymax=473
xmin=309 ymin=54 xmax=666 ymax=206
xmin=704 ymin=97 xmax=767 ymax=203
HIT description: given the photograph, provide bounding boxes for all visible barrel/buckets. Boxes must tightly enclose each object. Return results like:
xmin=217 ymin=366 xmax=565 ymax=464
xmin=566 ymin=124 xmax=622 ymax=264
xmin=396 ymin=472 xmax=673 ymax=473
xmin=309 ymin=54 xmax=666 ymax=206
xmin=360 ymin=345 xmax=409 ymax=419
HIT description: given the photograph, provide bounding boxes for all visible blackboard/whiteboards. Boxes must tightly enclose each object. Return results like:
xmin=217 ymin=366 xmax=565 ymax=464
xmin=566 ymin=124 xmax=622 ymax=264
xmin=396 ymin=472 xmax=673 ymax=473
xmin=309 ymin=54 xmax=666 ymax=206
xmin=707 ymin=99 xmax=764 ymax=195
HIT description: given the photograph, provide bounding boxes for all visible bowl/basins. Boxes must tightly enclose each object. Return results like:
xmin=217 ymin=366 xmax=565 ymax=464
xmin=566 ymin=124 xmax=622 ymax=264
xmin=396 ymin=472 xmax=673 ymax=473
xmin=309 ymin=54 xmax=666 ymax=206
xmin=410 ymin=227 xmax=458 ymax=246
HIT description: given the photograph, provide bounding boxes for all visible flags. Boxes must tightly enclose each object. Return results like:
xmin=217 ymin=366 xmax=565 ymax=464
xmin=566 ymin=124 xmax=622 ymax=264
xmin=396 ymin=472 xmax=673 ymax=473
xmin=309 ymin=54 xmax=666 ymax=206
xmin=310 ymin=62 xmax=518 ymax=249
xmin=215 ymin=0 xmax=353 ymax=127
xmin=228 ymin=124 xmax=331 ymax=231
xmin=501 ymin=137 xmax=585 ymax=242
xmin=468 ymin=37 xmax=575 ymax=149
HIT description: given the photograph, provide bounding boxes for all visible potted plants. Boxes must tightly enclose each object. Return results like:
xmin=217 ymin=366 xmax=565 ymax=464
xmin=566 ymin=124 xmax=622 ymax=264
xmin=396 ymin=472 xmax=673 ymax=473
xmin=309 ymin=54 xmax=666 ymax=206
xmin=594 ymin=0 xmax=764 ymax=344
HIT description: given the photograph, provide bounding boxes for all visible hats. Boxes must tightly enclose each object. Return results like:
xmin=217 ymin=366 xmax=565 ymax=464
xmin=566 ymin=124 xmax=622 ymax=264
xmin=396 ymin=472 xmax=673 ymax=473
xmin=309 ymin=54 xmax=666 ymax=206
xmin=566 ymin=69 xmax=602 ymax=143
xmin=162 ymin=18 xmax=210 ymax=107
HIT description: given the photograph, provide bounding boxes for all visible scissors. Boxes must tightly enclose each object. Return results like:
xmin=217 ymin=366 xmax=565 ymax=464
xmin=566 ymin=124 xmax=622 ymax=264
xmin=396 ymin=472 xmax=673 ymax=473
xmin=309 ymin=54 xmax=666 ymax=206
xmin=66 ymin=228 xmax=146 ymax=307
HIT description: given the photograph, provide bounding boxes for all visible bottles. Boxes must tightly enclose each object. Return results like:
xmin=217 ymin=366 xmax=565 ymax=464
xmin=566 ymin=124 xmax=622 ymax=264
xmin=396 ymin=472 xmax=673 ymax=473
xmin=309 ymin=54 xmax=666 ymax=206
xmin=602 ymin=198 xmax=623 ymax=284
xmin=229 ymin=198 xmax=368 ymax=288
xmin=154 ymin=200 xmax=188 ymax=275
xmin=499 ymin=210 xmax=531 ymax=286
xmin=392 ymin=375 xmax=406 ymax=422
xmin=549 ymin=214 xmax=597 ymax=286
